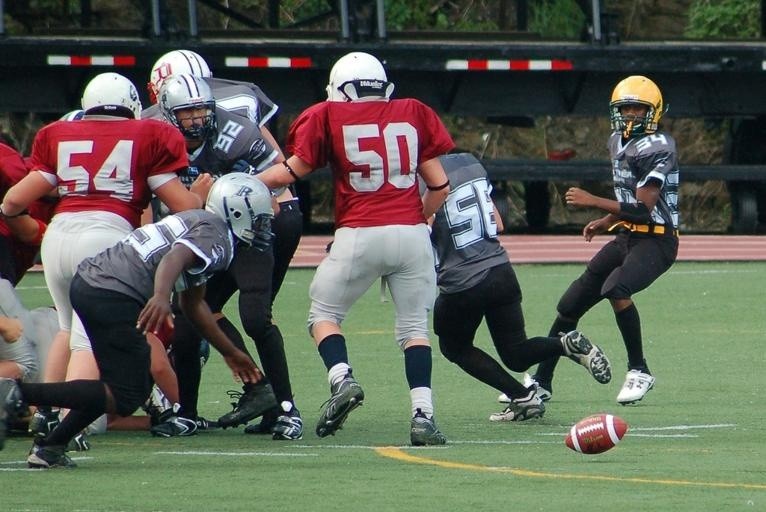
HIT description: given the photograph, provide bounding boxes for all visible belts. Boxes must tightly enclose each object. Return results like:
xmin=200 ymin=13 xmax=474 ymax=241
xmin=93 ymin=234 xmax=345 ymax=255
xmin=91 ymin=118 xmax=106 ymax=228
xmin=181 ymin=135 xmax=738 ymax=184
xmin=611 ymin=222 xmax=681 ymax=238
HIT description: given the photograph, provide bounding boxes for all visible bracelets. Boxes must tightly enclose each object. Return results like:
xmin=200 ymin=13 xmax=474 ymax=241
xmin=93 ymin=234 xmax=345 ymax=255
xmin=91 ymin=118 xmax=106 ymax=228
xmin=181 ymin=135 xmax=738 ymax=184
xmin=426 ymin=179 xmax=449 ymax=190
xmin=617 ymin=198 xmax=653 ymax=226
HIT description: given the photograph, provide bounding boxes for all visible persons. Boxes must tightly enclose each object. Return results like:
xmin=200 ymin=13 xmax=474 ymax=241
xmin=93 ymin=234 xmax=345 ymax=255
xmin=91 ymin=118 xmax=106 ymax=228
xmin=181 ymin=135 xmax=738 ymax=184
xmin=252 ymin=52 xmax=457 ymax=446
xmin=497 ymin=76 xmax=678 ymax=406
xmin=416 ymin=153 xmax=612 ymax=421
xmin=1 ymin=172 xmax=275 ymax=469
xmin=0 ymin=49 xmax=305 ymax=453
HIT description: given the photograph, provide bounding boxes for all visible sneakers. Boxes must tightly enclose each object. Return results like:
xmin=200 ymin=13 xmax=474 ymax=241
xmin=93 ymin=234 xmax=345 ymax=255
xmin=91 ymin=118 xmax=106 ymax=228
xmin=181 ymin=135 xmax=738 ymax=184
xmin=0 ymin=377 xmax=27 ymax=451
xmin=411 ymin=408 xmax=445 ymax=445
xmin=216 ymin=380 xmax=303 ymax=442
xmin=315 ymin=378 xmax=364 ymax=437
xmin=27 ymin=406 xmax=90 ymax=469
xmin=489 ymin=331 xmax=655 ymax=421
xmin=152 ymin=405 xmax=220 ymax=437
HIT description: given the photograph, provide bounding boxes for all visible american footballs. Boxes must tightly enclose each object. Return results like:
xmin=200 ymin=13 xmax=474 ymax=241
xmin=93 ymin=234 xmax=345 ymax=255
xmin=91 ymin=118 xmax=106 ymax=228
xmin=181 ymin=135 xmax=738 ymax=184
xmin=565 ymin=415 xmax=627 ymax=455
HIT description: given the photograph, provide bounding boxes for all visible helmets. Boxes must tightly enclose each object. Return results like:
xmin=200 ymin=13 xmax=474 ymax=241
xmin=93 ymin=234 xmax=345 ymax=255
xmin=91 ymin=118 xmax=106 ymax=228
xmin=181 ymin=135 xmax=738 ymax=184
xmin=326 ymin=51 xmax=395 ymax=103
xmin=203 ymin=174 xmax=275 ymax=253
xmin=81 ymin=71 xmax=142 ymax=120
xmin=146 ymin=50 xmax=213 ymax=106
xmin=157 ymin=74 xmax=217 ymax=138
xmin=609 ymin=75 xmax=671 ymax=134
